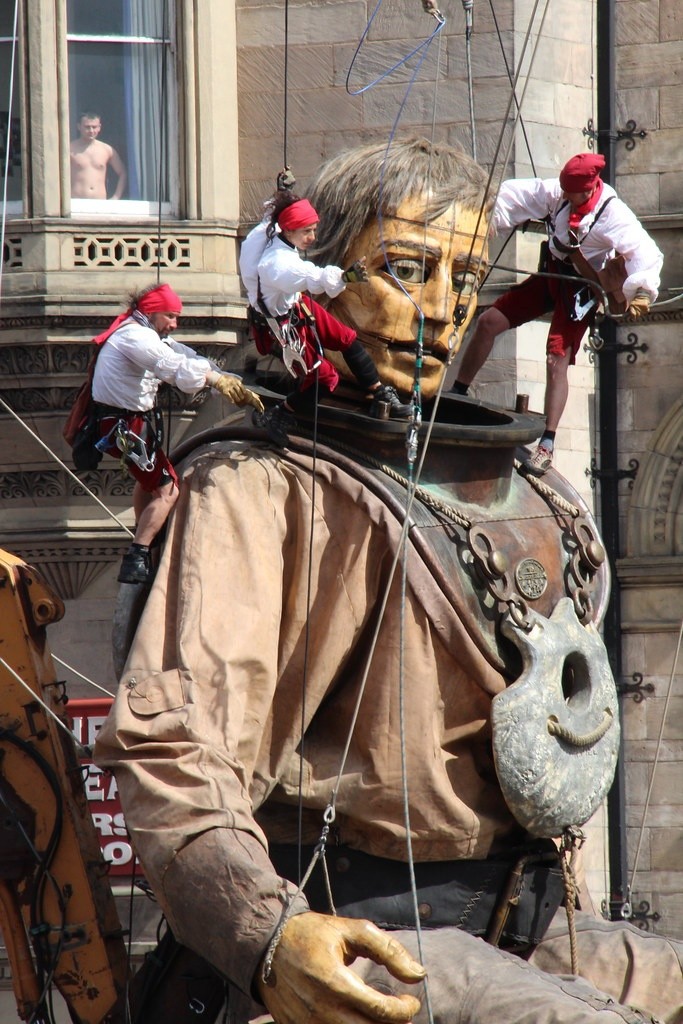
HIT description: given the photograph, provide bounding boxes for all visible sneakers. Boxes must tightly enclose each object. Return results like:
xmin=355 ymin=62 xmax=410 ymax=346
xmin=523 ymin=445 xmax=554 ymax=474
xmin=260 ymin=402 xmax=306 ymax=436
xmin=369 ymin=385 xmax=415 ymax=418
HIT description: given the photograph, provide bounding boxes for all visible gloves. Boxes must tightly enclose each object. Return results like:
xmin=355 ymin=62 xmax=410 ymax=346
xmin=238 ymin=388 xmax=265 ymax=413
xmin=624 ymin=288 xmax=652 ymax=321
xmin=213 ymin=373 xmax=246 ymax=404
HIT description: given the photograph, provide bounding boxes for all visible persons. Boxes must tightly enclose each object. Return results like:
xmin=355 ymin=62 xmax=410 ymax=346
xmin=92 ymin=134 xmax=683 ymax=1023
xmin=238 ymin=164 xmax=411 ymax=448
xmin=450 ymin=154 xmax=664 ymax=475
xmin=62 ymin=284 xmax=263 ymax=584
xmin=70 ymin=111 xmax=127 ymax=199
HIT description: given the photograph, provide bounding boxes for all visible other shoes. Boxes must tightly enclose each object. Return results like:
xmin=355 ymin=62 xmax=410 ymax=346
xmin=117 ymin=552 xmax=156 ymax=584
